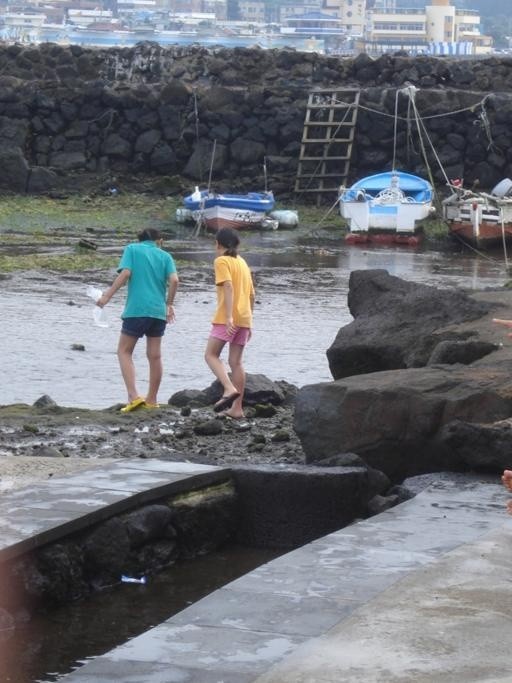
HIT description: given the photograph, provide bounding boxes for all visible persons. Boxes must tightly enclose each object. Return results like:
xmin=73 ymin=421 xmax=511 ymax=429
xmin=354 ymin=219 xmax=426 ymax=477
xmin=204 ymin=225 xmax=256 ymax=419
xmin=94 ymin=227 xmax=179 ymax=413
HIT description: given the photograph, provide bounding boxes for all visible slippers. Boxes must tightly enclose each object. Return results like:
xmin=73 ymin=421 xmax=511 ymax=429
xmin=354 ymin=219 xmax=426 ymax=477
xmin=212 ymin=390 xmax=244 ymax=421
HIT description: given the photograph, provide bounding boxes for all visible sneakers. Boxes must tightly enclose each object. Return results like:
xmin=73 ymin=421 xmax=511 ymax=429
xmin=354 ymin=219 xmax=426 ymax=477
xmin=139 ymin=402 xmax=161 ymax=410
xmin=117 ymin=397 xmax=144 ymax=413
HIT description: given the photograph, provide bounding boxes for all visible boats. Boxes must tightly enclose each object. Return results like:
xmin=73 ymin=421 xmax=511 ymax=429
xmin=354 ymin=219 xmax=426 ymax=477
xmin=340 ymin=172 xmax=512 ymax=249
xmin=183 ymin=189 xmax=275 ymax=232
